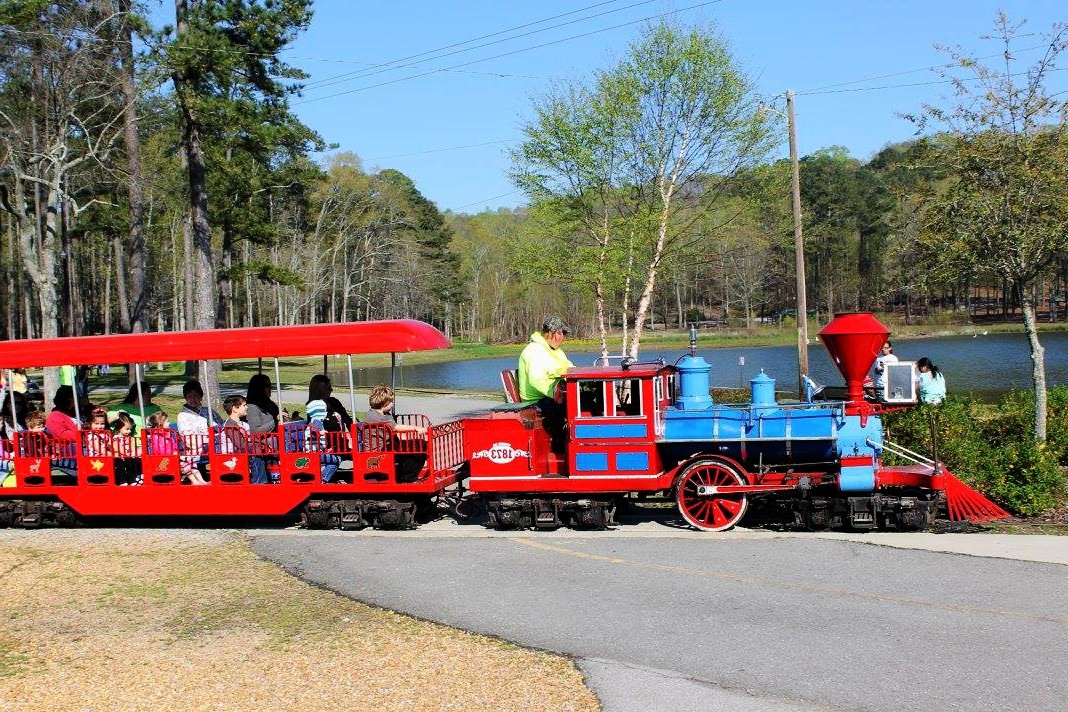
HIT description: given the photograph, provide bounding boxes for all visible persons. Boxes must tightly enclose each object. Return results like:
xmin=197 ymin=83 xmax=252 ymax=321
xmin=173 ymin=374 xmax=428 ymax=486
xmin=872 ymin=341 xmax=899 ymax=403
xmin=917 ymin=357 xmax=946 ymax=405
xmin=0 ymin=361 xmax=170 ymax=487
xmin=518 ymin=316 xmax=576 ymax=458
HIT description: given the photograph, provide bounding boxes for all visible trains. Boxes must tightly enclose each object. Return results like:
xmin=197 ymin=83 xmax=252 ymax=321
xmin=1 ymin=312 xmax=1012 ymax=531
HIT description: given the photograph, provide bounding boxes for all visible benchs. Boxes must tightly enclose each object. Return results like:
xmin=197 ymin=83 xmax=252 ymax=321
xmin=579 ymin=410 xmax=627 ymax=419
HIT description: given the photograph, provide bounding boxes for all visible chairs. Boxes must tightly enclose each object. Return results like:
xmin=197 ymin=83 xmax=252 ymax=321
xmin=500 ymin=368 xmax=521 ymax=406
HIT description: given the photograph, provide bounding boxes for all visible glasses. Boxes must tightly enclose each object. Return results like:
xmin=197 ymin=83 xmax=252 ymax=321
xmin=552 ymin=329 xmax=566 ymax=335
xmin=882 ymin=346 xmax=890 ymax=350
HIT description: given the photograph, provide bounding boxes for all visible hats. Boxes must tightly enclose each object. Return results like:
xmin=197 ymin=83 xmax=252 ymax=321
xmin=542 ymin=317 xmax=571 ymax=332
